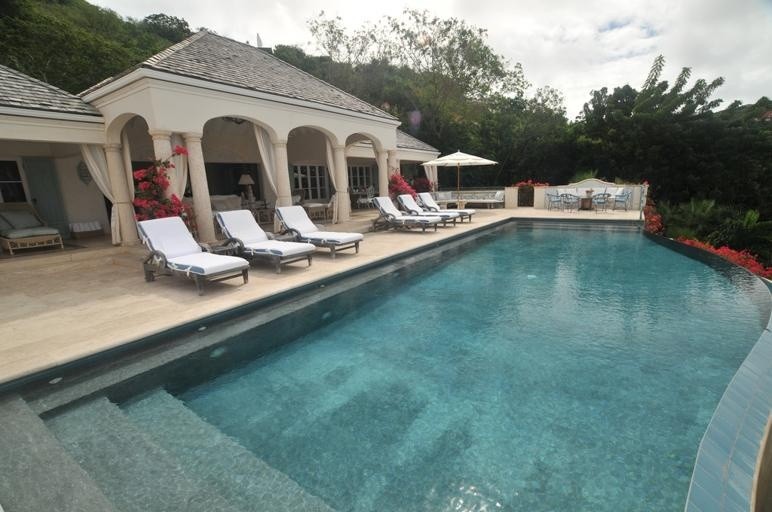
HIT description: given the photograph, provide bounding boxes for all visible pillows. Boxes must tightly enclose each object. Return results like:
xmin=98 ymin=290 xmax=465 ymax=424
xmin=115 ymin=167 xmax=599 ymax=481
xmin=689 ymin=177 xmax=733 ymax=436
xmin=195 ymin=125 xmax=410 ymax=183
xmin=303 ymin=202 xmax=328 ymax=207
xmin=0 ymin=225 xmax=59 ymax=239
xmin=494 ymin=191 xmax=505 ymax=200
xmin=1 ymin=208 xmax=44 ymax=230
xmin=437 ymin=191 xmax=451 ymax=200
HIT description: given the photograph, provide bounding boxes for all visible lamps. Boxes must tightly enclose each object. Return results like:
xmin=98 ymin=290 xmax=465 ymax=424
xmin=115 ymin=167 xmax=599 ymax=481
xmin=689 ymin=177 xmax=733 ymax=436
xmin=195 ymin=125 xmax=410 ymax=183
xmin=238 ymin=173 xmax=256 ymax=204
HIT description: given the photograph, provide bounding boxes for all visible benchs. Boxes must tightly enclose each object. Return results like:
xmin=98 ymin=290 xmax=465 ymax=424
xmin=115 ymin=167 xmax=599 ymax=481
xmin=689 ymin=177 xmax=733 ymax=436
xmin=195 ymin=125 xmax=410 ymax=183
xmin=433 ymin=199 xmax=505 ymax=209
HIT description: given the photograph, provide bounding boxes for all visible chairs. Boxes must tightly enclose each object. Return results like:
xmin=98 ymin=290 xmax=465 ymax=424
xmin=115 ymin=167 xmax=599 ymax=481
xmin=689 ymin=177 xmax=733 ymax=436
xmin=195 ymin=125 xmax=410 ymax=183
xmin=274 ymin=205 xmax=364 ymax=260
xmin=215 ymin=209 xmax=317 ymax=273
xmin=136 ymin=216 xmax=250 ymax=297
xmin=325 ymin=194 xmax=335 ymax=220
xmin=545 ymin=188 xmax=632 ymax=214
xmin=0 ymin=201 xmax=65 ymax=255
xmin=397 ymin=193 xmax=460 ymax=228
xmin=417 ymin=192 xmax=476 ymax=223
xmin=356 ymin=185 xmax=375 ymax=209
xmin=368 ymin=196 xmax=442 ymax=233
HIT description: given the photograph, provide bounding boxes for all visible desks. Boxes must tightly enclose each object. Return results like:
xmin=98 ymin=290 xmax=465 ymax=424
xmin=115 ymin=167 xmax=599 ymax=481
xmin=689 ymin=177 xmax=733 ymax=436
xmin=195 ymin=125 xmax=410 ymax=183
xmin=251 ymin=208 xmax=274 ymax=223
xmin=303 ymin=207 xmax=326 ymax=220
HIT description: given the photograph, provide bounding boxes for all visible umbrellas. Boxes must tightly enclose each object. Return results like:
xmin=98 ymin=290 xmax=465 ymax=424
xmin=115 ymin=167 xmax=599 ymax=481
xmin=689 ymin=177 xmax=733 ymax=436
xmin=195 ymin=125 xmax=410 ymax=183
xmin=421 ymin=149 xmax=499 ymax=209
